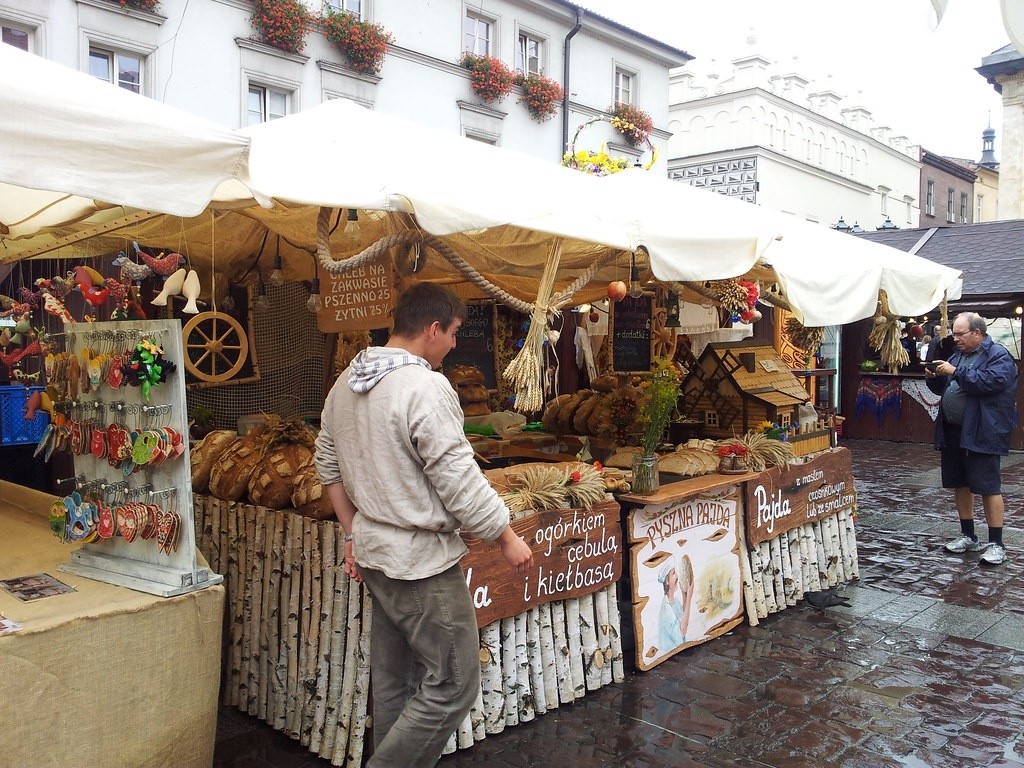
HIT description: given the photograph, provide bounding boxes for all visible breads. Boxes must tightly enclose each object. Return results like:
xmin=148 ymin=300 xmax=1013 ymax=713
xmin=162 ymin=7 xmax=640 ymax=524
xmin=655 ymin=448 xmax=722 ymax=477
xmin=604 ymin=446 xmax=639 ymax=468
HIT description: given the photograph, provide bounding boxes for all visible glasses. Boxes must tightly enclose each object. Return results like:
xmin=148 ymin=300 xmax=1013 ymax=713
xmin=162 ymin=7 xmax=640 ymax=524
xmin=952 ymin=329 xmax=974 ymax=338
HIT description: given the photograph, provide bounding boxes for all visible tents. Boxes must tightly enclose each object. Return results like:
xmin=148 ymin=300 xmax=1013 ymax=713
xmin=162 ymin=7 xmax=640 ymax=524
xmin=220 ymin=98 xmax=784 ymax=280
xmin=604 ymin=166 xmax=965 ymax=328
xmin=0 ymin=42 xmax=269 ymax=272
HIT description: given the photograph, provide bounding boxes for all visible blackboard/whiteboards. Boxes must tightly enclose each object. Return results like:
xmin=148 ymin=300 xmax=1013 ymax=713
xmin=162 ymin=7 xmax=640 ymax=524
xmin=607 ymin=290 xmax=659 ymax=377
xmin=437 ymin=298 xmax=502 ymax=395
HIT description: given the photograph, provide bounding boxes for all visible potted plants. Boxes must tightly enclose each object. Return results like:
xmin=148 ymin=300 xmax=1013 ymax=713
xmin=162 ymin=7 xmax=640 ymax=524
xmin=632 ymin=357 xmax=684 ymax=495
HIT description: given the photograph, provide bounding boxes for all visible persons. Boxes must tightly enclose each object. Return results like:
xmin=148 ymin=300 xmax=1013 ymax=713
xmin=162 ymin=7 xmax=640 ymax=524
xmin=313 ymin=283 xmax=533 ymax=768
xmin=899 ymin=324 xmax=957 ymax=365
xmin=926 ymin=312 xmax=1018 ymax=565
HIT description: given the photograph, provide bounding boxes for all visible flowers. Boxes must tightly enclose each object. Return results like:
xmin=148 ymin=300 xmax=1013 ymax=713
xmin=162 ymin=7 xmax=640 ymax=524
xmin=612 ymin=101 xmax=653 ymax=146
xmin=757 ymin=419 xmax=800 ymax=442
xmin=112 ymin=0 xmax=161 ymax=15
xmin=456 ymin=48 xmax=513 ymax=104
xmin=245 ymin=0 xmax=312 ymax=53
xmin=512 ymin=67 xmax=565 ymax=123
xmin=312 ymin=0 xmax=395 ymax=77
xmin=718 ymin=443 xmax=748 ymax=456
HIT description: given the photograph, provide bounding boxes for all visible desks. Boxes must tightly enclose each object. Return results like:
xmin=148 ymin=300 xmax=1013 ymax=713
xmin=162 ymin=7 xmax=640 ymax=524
xmin=619 ymin=471 xmax=759 ymax=672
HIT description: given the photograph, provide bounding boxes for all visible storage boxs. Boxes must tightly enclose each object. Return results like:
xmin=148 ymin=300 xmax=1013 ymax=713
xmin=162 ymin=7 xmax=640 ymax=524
xmin=0 ymin=386 xmax=48 ymax=446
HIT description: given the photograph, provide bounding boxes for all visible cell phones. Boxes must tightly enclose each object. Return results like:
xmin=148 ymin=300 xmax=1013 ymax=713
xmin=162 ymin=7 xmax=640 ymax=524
xmin=925 ymin=363 xmax=940 ymax=373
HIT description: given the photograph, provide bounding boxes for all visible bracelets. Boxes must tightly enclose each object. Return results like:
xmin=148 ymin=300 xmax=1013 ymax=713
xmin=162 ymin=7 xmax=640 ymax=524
xmin=345 ymin=535 xmax=352 ymax=542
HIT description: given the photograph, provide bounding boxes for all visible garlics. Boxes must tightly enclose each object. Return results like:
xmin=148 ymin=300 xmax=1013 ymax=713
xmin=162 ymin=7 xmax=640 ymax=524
xmin=875 ymin=316 xmax=887 ymax=325
xmin=741 ymin=310 xmax=762 ymax=324
xmin=549 ymin=330 xmax=560 ymax=342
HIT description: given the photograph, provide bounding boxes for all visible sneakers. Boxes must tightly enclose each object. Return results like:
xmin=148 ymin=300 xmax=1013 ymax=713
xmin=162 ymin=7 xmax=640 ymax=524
xmin=979 ymin=543 xmax=1007 ymax=564
xmin=943 ymin=534 xmax=980 ymax=553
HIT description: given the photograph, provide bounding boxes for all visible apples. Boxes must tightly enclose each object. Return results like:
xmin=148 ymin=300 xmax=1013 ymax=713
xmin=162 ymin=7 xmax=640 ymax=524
xmin=607 ymin=280 xmax=627 ymax=302
xmin=911 ymin=324 xmax=923 ymax=336
xmin=604 ymin=477 xmax=631 ymax=493
xmin=589 ymin=313 xmax=599 ymax=322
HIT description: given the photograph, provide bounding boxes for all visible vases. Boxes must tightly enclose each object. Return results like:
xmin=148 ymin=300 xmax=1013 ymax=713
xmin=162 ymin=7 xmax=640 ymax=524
xmin=720 ymin=456 xmax=749 ymax=474
